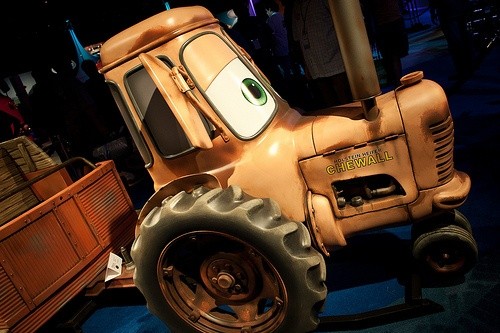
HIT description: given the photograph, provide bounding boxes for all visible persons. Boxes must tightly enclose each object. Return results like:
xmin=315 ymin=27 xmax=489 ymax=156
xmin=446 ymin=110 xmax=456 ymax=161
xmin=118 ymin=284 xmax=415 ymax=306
xmin=264 ymin=1 xmax=303 ymax=77
xmin=80 ymin=60 xmax=149 ymax=184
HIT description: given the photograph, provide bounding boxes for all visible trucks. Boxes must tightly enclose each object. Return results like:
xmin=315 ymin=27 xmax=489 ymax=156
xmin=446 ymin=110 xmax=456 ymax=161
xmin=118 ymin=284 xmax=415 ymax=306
xmin=0 ymin=7 xmax=477 ymax=333
